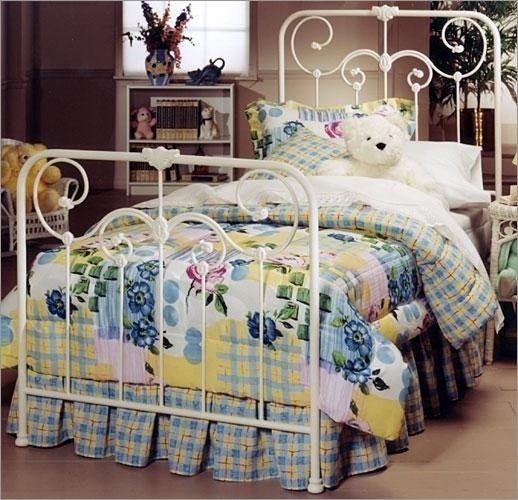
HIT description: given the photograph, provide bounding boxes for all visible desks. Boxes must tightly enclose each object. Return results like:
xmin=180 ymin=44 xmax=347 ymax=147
xmin=483 ymin=196 xmax=515 ymax=366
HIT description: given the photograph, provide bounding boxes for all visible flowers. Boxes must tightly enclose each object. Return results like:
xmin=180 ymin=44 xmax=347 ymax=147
xmin=118 ymin=0 xmax=203 ymax=72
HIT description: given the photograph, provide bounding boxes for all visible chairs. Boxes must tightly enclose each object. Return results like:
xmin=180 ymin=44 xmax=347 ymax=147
xmin=0 ymin=137 xmax=81 ymax=260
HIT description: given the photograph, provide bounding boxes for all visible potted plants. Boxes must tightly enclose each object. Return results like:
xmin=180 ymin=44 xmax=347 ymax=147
xmin=429 ymin=0 xmax=518 ymax=151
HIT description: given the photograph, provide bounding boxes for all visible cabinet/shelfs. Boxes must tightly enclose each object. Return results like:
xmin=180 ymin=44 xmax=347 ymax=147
xmin=123 ymin=81 xmax=236 ymax=198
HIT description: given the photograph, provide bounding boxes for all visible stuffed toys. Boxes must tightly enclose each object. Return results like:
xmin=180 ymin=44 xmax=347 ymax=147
xmin=130 ymin=106 xmax=156 ymax=139
xmin=198 ymin=107 xmax=220 ymax=140
xmin=321 ymin=112 xmax=437 ymax=195
xmin=0 ymin=141 xmax=61 ymax=214
xmin=498 ymin=236 xmax=517 ymax=297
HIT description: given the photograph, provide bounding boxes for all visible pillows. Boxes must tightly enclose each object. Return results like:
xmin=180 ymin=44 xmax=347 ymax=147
xmin=400 ymin=137 xmax=489 ymax=209
xmin=244 ymin=96 xmax=416 ymax=160
xmin=249 ymin=125 xmax=349 ymax=179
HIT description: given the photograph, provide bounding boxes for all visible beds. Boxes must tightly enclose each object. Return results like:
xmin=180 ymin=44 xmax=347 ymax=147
xmin=13 ymin=7 xmax=503 ymax=493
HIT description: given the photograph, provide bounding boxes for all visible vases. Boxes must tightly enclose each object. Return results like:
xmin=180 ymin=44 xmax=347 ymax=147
xmin=145 ymin=47 xmax=174 ymax=84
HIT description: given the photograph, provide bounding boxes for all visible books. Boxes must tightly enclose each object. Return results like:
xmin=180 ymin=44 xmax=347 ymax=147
xmin=155 ymin=100 xmax=201 ymax=139
xmin=129 ymin=143 xmax=180 ymax=183
xmin=182 ymin=173 xmax=227 ymax=182
